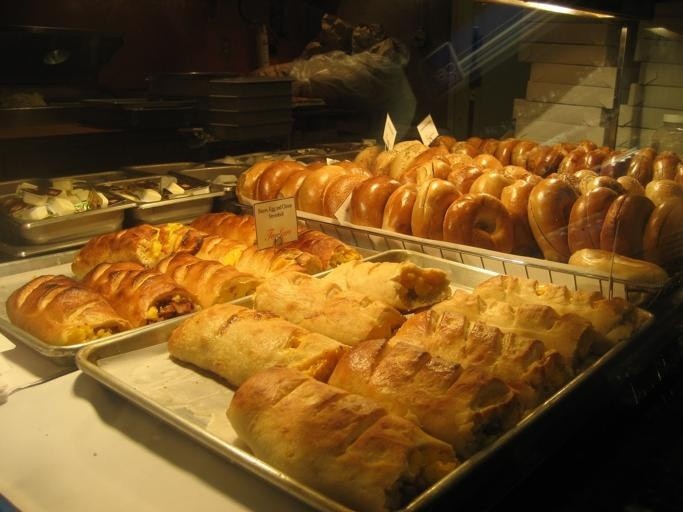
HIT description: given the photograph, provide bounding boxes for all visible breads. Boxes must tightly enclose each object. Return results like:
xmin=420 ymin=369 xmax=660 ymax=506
xmin=0 ymin=135 xmax=683 ymax=512
